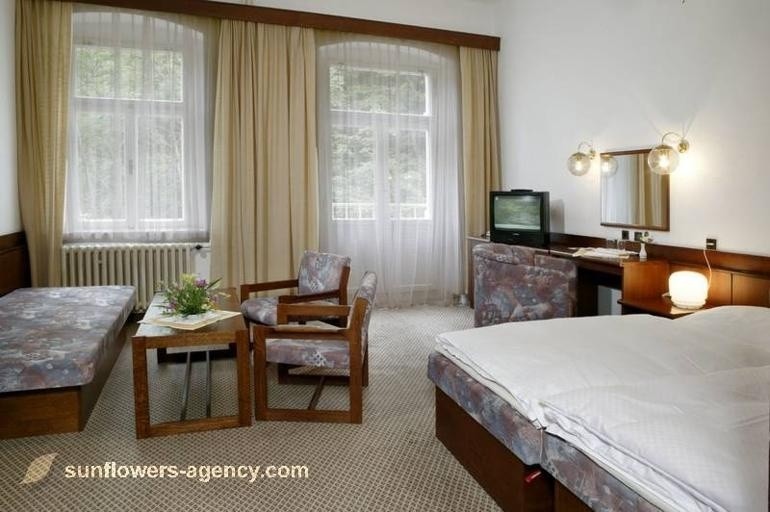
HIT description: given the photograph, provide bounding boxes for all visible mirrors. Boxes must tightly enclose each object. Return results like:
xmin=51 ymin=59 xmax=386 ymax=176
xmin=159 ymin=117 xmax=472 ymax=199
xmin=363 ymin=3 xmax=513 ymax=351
xmin=601 ymin=149 xmax=670 ymax=232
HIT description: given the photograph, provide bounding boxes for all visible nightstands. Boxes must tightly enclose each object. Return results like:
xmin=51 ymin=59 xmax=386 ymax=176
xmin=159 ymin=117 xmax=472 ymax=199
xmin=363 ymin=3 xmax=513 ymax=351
xmin=616 ymin=299 xmax=717 ymax=320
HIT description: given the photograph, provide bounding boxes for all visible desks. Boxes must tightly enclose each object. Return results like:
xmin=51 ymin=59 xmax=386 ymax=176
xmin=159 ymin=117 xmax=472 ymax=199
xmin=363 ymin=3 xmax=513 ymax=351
xmin=465 ymin=233 xmax=667 ymax=309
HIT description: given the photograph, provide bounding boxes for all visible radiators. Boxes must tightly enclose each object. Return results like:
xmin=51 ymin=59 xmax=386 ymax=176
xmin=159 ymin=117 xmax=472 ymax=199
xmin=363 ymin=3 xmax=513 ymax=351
xmin=61 ymin=244 xmax=204 ymax=314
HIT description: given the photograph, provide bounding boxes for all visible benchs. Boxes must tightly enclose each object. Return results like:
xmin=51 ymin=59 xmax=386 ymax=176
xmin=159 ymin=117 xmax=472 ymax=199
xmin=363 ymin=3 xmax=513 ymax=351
xmin=0 ymin=231 xmax=136 ymax=440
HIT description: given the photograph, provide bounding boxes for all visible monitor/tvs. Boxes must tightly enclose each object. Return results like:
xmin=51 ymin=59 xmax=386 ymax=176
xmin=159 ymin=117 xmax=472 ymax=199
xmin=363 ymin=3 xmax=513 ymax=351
xmin=489 ymin=191 xmax=551 ymax=249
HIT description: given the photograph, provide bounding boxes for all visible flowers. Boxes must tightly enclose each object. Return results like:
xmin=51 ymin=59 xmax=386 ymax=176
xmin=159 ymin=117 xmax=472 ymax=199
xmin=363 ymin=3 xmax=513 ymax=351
xmin=151 ymin=273 xmax=223 ymax=318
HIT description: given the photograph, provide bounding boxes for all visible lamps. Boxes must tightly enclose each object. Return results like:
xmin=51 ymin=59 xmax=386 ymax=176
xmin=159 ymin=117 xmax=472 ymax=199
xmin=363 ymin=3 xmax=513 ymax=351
xmin=599 ymin=155 xmax=618 ymax=179
xmin=647 ymin=132 xmax=689 ymax=175
xmin=568 ymin=141 xmax=596 ymax=175
xmin=668 ymin=250 xmax=713 ymax=308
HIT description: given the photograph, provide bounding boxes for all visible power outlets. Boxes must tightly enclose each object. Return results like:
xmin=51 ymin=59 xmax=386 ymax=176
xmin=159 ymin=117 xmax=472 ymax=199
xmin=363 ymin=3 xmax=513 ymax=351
xmin=704 ymin=238 xmax=718 ymax=251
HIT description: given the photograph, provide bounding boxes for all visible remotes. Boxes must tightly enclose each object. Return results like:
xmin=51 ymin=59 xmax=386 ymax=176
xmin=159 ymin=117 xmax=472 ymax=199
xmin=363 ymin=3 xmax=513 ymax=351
xmin=511 ymin=189 xmax=533 ymax=192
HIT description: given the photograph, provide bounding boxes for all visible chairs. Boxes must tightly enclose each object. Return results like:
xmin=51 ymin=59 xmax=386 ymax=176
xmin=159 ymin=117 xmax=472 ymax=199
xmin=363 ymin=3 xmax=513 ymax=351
xmin=472 ymin=242 xmax=577 ymax=328
xmin=241 ymin=251 xmax=351 ymax=352
xmin=253 ymin=270 xmax=377 ymax=424
